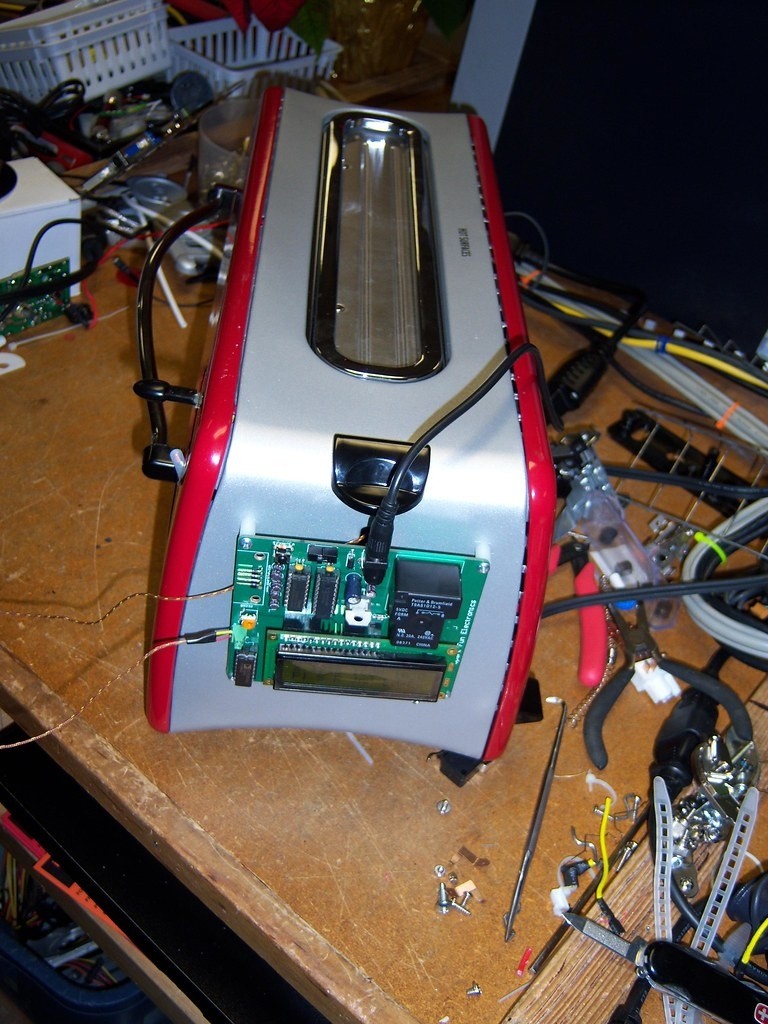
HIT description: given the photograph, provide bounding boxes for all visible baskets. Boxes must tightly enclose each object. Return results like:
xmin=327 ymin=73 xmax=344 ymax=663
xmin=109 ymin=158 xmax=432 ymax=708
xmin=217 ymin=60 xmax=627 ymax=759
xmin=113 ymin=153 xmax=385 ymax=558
xmin=0 ymin=1 xmax=175 ymax=108
xmin=163 ymin=10 xmax=345 ymax=123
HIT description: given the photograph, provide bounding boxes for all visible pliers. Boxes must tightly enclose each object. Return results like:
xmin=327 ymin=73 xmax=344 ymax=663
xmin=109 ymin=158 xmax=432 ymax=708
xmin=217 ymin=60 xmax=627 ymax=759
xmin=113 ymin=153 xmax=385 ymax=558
xmin=547 ymin=530 xmax=606 ymax=687
xmin=583 ymin=600 xmax=755 ymax=769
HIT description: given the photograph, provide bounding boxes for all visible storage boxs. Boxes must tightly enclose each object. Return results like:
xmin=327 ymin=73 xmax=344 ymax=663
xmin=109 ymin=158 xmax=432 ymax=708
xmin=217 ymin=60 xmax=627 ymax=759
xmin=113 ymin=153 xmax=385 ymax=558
xmin=0 ymin=0 xmax=173 ymax=118
xmin=153 ymin=13 xmax=345 ymax=104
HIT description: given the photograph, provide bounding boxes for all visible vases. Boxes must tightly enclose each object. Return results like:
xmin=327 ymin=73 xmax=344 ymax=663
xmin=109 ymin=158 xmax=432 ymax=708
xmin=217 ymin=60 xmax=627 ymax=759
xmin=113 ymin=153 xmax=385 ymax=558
xmin=327 ymin=0 xmax=431 ymax=73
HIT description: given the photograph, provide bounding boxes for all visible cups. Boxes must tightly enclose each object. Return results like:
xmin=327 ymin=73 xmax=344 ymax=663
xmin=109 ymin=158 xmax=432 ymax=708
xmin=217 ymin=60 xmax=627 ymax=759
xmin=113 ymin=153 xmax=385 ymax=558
xmin=198 ymin=97 xmax=261 ymax=208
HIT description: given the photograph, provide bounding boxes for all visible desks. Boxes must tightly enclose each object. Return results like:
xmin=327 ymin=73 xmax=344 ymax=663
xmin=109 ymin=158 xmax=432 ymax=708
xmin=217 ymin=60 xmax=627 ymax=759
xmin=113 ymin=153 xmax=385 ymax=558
xmin=0 ymin=105 xmax=767 ymax=1021
